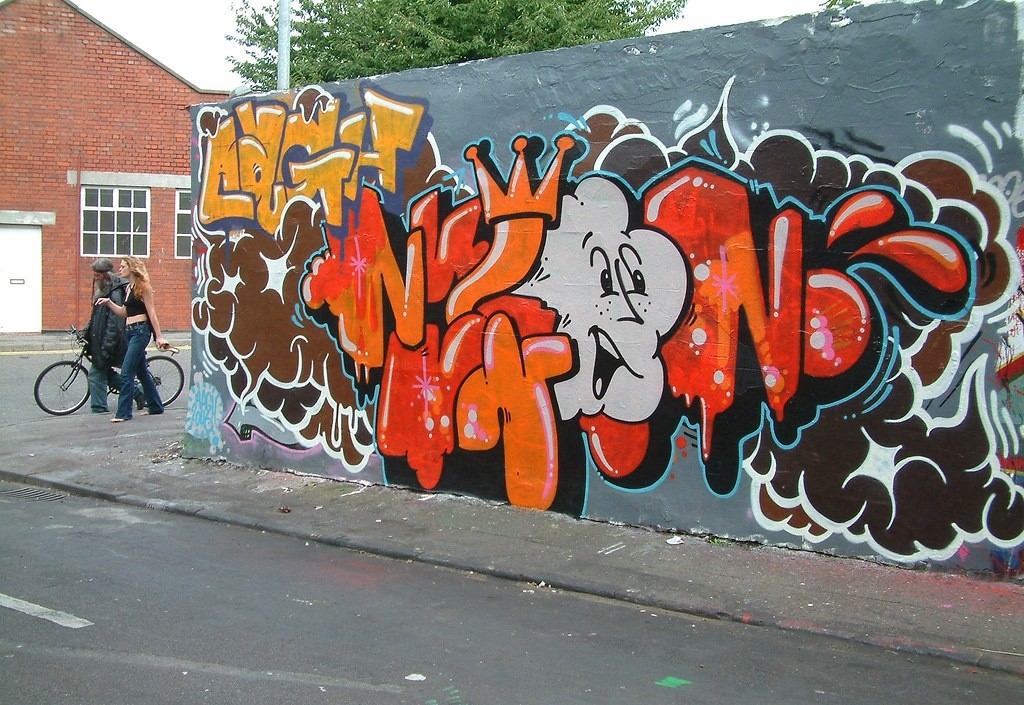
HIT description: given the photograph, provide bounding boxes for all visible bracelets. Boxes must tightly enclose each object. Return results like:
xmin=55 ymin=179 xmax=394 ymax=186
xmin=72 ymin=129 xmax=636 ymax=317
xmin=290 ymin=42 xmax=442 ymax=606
xmin=105 ymin=298 xmax=110 ymax=305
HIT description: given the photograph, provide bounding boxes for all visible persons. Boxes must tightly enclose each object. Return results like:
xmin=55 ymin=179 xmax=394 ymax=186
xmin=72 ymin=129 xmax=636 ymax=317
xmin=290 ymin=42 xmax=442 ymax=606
xmin=78 ymin=256 xmax=169 ymax=423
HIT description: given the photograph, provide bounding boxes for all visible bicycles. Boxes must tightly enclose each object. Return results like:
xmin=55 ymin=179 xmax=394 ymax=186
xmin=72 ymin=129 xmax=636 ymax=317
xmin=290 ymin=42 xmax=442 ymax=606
xmin=33 ymin=324 xmax=185 ymax=416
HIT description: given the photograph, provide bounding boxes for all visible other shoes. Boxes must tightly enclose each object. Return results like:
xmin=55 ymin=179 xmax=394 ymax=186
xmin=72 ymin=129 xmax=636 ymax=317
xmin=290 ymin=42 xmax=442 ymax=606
xmin=135 ymin=391 xmax=145 ymax=410
xmin=91 ymin=407 xmax=110 ymax=414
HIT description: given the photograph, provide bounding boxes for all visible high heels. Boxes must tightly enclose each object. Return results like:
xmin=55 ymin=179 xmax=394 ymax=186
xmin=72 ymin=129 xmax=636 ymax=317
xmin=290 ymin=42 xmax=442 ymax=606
xmin=156 ymin=343 xmax=180 ymax=355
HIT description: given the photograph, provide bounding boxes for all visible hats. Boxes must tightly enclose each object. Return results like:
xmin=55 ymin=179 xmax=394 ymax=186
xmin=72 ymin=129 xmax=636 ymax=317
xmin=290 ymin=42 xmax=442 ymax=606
xmin=92 ymin=258 xmax=113 ymax=273
